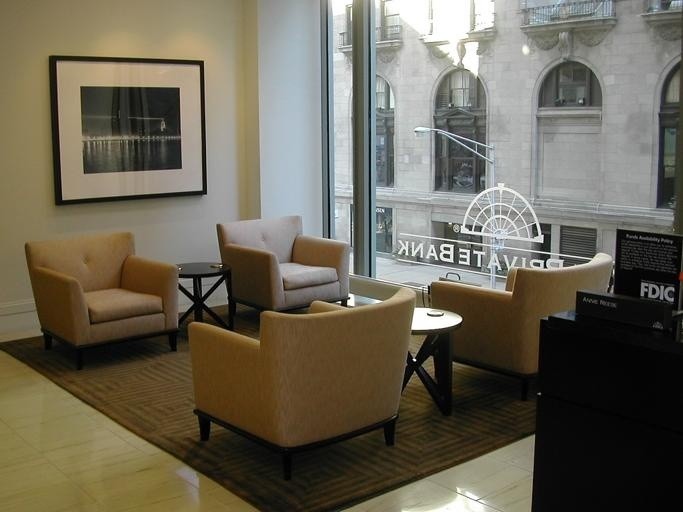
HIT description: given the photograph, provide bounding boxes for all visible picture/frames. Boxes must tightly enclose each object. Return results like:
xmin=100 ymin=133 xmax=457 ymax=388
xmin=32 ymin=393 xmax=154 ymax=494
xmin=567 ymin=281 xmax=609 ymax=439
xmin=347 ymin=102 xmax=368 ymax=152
xmin=47 ymin=53 xmax=209 ymax=208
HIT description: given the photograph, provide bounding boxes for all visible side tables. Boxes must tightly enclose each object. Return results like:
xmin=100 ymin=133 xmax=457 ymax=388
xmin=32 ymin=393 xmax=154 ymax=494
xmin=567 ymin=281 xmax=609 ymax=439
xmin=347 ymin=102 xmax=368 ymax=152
xmin=402 ymin=307 xmax=464 ymax=417
xmin=173 ymin=261 xmax=234 ymax=332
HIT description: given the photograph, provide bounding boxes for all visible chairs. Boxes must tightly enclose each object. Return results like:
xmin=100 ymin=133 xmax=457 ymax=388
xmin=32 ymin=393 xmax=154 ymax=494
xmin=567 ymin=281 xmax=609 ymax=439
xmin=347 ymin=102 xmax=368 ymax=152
xmin=215 ymin=215 xmax=352 ymax=317
xmin=24 ymin=230 xmax=179 ymax=370
xmin=429 ymin=251 xmax=614 ymax=403
xmin=187 ymin=286 xmax=416 ymax=483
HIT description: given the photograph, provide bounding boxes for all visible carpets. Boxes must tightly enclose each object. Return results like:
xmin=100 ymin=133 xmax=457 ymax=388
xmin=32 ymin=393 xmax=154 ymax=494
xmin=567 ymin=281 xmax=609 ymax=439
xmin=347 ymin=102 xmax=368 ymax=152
xmin=0 ymin=299 xmax=536 ymax=512
